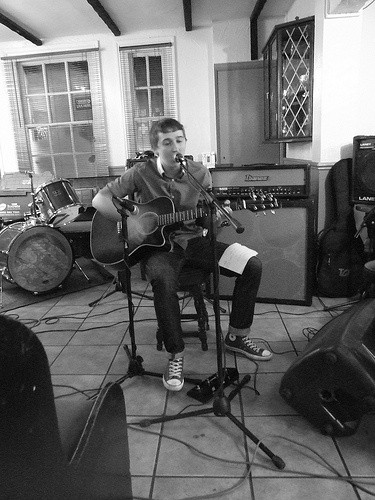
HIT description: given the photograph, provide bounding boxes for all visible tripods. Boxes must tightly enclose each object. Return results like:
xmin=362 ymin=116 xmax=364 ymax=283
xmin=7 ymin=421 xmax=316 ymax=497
xmin=139 ymin=161 xmax=288 ymax=470
xmin=87 ymin=209 xmax=203 ymax=401
xmin=88 ymin=270 xmax=154 ymax=307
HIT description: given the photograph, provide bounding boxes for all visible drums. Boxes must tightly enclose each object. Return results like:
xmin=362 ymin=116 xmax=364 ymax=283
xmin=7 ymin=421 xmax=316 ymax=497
xmin=0 ymin=221 xmax=75 ymax=295
xmin=34 ymin=177 xmax=84 ymax=228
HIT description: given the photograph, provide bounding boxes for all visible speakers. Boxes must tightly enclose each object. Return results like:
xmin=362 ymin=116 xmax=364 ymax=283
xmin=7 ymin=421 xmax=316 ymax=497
xmin=207 ymin=197 xmax=319 ymax=306
xmin=281 ymin=299 xmax=375 ymax=437
xmin=350 ymin=135 xmax=375 ymax=206
xmin=206 ymin=163 xmax=320 ymax=306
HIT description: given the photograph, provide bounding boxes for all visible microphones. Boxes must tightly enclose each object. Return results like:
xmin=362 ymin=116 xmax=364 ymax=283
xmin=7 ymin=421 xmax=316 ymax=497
xmin=113 ymin=196 xmax=139 ymax=216
xmin=173 ymin=152 xmax=184 ymax=162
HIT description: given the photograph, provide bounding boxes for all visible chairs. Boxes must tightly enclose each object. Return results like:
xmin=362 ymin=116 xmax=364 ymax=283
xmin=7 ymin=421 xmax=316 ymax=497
xmin=0 ymin=316 xmax=133 ymax=500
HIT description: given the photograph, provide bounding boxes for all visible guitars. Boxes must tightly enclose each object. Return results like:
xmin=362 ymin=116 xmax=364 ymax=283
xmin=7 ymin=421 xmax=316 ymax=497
xmin=90 ymin=192 xmax=280 ymax=269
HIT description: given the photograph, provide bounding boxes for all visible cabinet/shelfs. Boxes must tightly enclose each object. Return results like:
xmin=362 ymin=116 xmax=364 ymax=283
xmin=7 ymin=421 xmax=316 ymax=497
xmin=259 ymin=16 xmax=315 ymax=144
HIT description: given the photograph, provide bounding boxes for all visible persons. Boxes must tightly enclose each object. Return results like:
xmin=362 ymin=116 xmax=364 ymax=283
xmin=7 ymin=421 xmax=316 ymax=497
xmin=92 ymin=118 xmax=272 ymax=391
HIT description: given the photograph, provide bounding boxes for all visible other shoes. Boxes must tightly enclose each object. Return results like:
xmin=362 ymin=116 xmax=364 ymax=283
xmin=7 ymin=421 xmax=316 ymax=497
xmin=162 ymin=356 xmax=185 ymax=392
xmin=224 ymin=333 xmax=273 ymax=361
